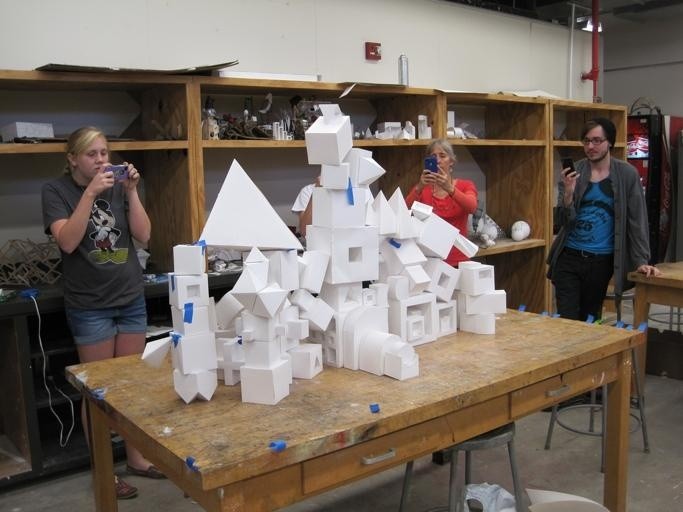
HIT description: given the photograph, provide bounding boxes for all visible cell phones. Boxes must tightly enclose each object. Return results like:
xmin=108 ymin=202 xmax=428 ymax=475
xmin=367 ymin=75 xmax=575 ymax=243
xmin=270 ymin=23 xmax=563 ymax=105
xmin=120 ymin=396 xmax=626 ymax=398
xmin=563 ymin=157 xmax=574 ymax=175
xmin=424 ymin=157 xmax=437 ymax=172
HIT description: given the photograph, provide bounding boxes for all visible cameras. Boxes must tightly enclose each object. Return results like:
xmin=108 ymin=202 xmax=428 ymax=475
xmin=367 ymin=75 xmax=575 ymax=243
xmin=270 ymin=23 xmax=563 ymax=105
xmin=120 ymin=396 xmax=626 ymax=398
xmin=106 ymin=165 xmax=128 ymax=181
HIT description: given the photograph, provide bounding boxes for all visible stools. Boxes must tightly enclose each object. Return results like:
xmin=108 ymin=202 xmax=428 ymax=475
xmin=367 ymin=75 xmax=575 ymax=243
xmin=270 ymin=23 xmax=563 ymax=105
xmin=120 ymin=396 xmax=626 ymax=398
xmin=648 ymin=299 xmax=682 ymax=332
xmin=397 ymin=420 xmax=532 ymax=511
xmin=600 ymin=291 xmax=635 ymax=325
xmin=544 ymin=345 xmax=652 ymax=475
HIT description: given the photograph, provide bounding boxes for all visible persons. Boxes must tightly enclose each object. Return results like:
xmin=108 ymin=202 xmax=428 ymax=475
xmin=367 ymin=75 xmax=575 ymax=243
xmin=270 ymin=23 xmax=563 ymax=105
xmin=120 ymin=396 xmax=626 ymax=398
xmin=405 ymin=137 xmax=478 ymax=269
xmin=42 ymin=127 xmax=165 ymax=500
xmin=539 ymin=115 xmax=663 ymax=413
xmin=290 ymin=173 xmax=321 ymax=252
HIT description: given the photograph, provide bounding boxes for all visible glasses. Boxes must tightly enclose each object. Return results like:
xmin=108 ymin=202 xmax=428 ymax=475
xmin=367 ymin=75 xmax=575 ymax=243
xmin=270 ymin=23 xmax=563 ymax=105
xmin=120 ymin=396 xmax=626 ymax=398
xmin=581 ymin=137 xmax=607 ymax=146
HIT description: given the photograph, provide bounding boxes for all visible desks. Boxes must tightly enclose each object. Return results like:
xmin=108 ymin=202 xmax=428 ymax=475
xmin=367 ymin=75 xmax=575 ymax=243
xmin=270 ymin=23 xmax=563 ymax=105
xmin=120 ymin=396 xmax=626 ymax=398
xmin=628 ymin=260 xmax=683 ymax=381
xmin=61 ymin=309 xmax=648 ymax=510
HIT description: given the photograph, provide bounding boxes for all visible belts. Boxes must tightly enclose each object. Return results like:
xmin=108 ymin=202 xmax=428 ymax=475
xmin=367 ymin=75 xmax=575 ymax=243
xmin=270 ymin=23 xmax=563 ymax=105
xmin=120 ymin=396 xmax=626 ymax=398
xmin=562 ymin=246 xmax=613 ymax=259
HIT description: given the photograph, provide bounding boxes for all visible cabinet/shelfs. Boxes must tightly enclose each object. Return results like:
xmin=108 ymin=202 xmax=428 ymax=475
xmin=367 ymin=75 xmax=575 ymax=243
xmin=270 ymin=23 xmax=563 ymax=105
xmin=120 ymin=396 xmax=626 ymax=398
xmin=0 ymin=259 xmax=170 ymax=493
xmin=549 ymin=101 xmax=628 ymax=166
xmin=0 ymin=67 xmax=204 ymax=276
xmin=193 ymin=74 xmax=444 ymax=270
xmin=441 ymin=93 xmax=550 ymax=314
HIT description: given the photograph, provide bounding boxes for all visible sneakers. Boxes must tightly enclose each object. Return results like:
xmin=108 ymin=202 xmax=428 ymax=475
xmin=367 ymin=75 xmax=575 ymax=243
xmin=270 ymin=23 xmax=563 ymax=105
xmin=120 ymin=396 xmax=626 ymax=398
xmin=540 ymin=386 xmax=602 ymax=412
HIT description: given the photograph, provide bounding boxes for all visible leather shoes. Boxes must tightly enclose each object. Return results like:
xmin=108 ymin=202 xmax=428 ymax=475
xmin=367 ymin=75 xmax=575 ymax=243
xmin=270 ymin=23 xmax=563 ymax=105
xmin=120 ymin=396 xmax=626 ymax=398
xmin=125 ymin=462 xmax=168 ymax=480
xmin=112 ymin=473 xmax=138 ymax=499
